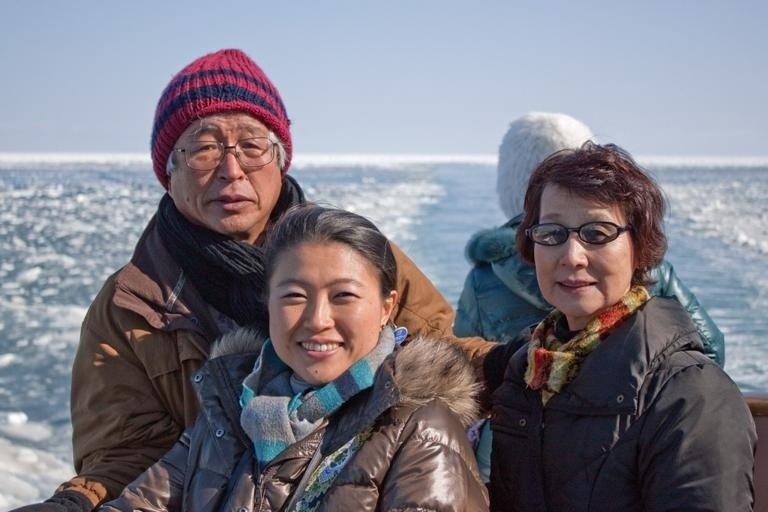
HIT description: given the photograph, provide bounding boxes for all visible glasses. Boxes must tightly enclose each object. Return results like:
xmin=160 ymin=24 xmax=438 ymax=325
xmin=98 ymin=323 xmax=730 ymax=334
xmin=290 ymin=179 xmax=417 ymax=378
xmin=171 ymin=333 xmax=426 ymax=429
xmin=524 ymin=222 xmax=631 ymax=246
xmin=169 ymin=137 xmax=276 ymax=171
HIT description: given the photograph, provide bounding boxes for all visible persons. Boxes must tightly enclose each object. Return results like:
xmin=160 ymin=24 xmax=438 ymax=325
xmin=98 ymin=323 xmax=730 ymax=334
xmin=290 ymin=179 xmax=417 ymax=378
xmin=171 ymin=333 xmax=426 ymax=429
xmin=487 ymin=138 xmax=761 ymax=511
xmin=451 ymin=111 xmax=726 ymax=489
xmin=91 ymin=198 xmax=490 ymax=512
xmin=7 ymin=49 xmax=543 ymax=511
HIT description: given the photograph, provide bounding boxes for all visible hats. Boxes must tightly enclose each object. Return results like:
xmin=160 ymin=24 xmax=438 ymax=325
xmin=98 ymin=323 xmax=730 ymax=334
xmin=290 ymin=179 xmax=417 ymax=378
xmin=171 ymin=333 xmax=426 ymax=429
xmin=148 ymin=47 xmax=292 ymax=191
